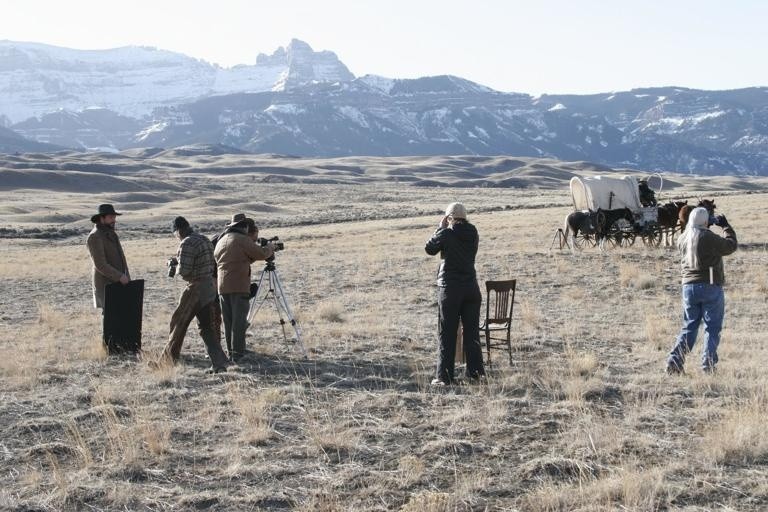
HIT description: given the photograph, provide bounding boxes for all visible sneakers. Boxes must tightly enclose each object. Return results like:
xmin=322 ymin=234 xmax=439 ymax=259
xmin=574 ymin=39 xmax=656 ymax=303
xmin=430 ymin=379 xmax=450 ymax=386
xmin=206 ymin=368 xmax=226 ymax=373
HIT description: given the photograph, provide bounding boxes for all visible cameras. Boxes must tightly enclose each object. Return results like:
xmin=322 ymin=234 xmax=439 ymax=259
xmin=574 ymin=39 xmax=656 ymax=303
xmin=167 ymin=259 xmax=178 ymax=278
xmin=709 ymin=217 xmax=720 ymax=224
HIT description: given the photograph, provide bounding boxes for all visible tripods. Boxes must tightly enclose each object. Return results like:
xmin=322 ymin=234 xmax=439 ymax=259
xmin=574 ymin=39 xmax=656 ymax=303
xmin=245 ymin=262 xmax=308 ymax=360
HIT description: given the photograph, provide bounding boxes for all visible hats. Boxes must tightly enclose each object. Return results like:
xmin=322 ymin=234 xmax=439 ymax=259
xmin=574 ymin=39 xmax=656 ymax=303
xmin=92 ymin=204 xmax=122 ymax=222
xmin=171 ymin=216 xmax=188 ymax=232
xmin=446 ymin=203 xmax=467 ymax=221
xmin=228 ymin=213 xmax=253 ymax=227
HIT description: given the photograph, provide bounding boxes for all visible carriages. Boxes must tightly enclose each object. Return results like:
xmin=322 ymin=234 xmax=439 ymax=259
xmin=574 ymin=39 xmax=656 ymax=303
xmin=567 ymin=170 xmax=715 ymax=250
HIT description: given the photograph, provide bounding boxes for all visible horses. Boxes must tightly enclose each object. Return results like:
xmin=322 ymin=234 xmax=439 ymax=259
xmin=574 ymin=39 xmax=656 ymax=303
xmin=561 ymin=206 xmax=635 ymax=256
xmin=657 ymin=200 xmax=688 ymax=246
xmin=678 ymin=199 xmax=717 ymax=234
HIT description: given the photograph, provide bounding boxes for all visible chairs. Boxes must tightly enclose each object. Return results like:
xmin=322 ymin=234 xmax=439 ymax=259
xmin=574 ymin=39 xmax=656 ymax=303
xmin=462 ymin=280 xmax=515 ymax=367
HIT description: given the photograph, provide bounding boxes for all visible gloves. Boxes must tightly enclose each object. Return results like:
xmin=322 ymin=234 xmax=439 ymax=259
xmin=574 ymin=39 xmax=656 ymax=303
xmin=714 ymin=215 xmax=728 ymax=226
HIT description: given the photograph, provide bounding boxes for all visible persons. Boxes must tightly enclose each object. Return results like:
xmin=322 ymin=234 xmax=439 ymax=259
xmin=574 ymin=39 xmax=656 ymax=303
xmin=425 ymin=202 xmax=487 ymax=386
xmin=664 ymin=207 xmax=737 ymax=377
xmin=213 ymin=213 xmax=281 ymax=363
xmin=204 ymin=225 xmax=258 ymax=359
xmin=146 ymin=216 xmax=233 ymax=374
xmin=86 ymin=203 xmax=132 ymax=316
